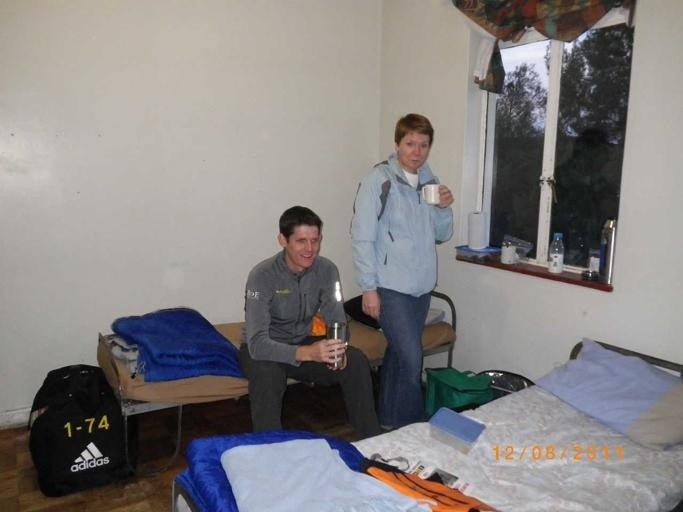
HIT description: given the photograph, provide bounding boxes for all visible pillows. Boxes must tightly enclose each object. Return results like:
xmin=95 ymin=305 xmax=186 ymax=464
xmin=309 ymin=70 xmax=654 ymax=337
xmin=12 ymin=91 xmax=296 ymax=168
xmin=532 ymin=339 xmax=683 ymax=449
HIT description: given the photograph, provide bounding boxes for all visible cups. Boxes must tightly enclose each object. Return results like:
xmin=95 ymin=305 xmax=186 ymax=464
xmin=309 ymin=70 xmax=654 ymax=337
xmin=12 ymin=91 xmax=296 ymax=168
xmin=325 ymin=321 xmax=346 ymax=369
xmin=421 ymin=183 xmax=441 ymax=206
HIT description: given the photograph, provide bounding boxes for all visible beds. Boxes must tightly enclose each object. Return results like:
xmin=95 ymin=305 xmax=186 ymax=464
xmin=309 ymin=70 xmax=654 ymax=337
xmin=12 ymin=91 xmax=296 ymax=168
xmin=171 ymin=338 xmax=683 ymax=511
xmin=95 ymin=276 xmax=460 ymax=481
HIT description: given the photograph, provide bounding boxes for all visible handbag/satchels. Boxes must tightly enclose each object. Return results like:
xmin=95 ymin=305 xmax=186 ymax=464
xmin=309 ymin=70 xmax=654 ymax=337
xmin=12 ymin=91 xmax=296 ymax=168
xmin=424 ymin=366 xmax=497 ymax=420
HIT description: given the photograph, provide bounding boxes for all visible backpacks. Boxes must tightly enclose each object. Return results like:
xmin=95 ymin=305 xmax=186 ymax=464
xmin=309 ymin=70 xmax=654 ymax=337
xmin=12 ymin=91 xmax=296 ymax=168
xmin=25 ymin=362 xmax=127 ymax=499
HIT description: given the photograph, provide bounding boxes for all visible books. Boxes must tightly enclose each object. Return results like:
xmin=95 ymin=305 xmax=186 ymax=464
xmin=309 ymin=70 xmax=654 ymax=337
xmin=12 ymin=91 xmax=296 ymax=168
xmin=408 ymin=459 xmax=471 ymax=496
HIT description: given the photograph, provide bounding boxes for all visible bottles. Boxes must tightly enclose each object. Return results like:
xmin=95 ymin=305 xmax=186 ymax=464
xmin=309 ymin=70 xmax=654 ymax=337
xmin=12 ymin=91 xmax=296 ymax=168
xmin=548 ymin=232 xmax=564 ymax=274
xmin=599 ymin=218 xmax=617 ymax=285
xmin=500 ymin=241 xmax=514 ymax=265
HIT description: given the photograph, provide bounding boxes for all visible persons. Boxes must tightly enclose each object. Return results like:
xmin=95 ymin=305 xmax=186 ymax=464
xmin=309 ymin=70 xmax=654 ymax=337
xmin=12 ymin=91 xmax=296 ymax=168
xmin=348 ymin=112 xmax=453 ymax=431
xmin=237 ymin=205 xmax=381 ymax=439
